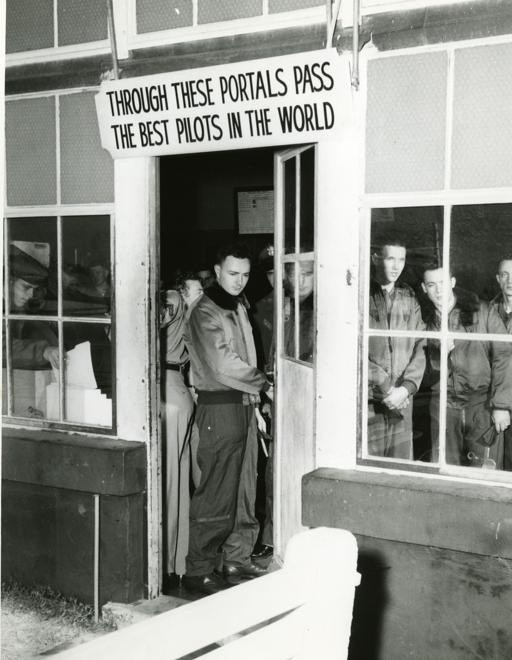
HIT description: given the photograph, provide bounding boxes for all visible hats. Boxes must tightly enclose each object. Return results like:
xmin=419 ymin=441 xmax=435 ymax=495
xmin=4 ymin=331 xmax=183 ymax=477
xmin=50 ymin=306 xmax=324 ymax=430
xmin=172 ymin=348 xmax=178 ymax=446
xmin=10 ymin=255 xmax=49 ymax=285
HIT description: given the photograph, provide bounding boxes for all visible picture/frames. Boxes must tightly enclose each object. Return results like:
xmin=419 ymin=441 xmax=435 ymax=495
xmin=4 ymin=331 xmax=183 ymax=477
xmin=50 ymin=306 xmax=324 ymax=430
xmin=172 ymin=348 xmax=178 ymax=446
xmin=235 ymin=186 xmax=296 ymax=238
xmin=371 ymin=208 xmax=397 ymax=229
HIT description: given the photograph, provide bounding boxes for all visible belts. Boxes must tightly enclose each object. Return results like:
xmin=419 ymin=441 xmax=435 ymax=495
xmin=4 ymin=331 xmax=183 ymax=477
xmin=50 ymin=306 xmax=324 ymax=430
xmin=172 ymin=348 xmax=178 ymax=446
xmin=164 ymin=362 xmax=186 ymax=373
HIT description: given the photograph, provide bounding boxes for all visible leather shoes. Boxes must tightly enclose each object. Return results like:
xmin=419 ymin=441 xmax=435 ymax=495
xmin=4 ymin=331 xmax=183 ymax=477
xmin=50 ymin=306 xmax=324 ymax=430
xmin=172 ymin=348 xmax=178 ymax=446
xmin=222 ymin=562 xmax=268 ymax=582
xmin=251 ymin=545 xmax=273 ymax=560
xmin=181 ymin=575 xmax=229 ymax=595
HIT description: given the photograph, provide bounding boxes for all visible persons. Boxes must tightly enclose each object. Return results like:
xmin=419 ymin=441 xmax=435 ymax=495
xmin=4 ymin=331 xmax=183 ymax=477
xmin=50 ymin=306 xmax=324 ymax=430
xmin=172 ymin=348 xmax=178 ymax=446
xmin=247 ymin=245 xmax=274 ymax=564
xmin=418 ymin=259 xmax=512 ymax=472
xmin=364 ymin=237 xmax=429 ymax=463
xmin=158 ymin=267 xmax=217 ymax=584
xmin=3 ymin=242 xmax=113 ymax=427
xmin=486 ymin=259 xmax=512 ymax=352
xmin=181 ymin=244 xmax=270 ymax=598
xmin=284 ymin=246 xmax=313 ymax=365
